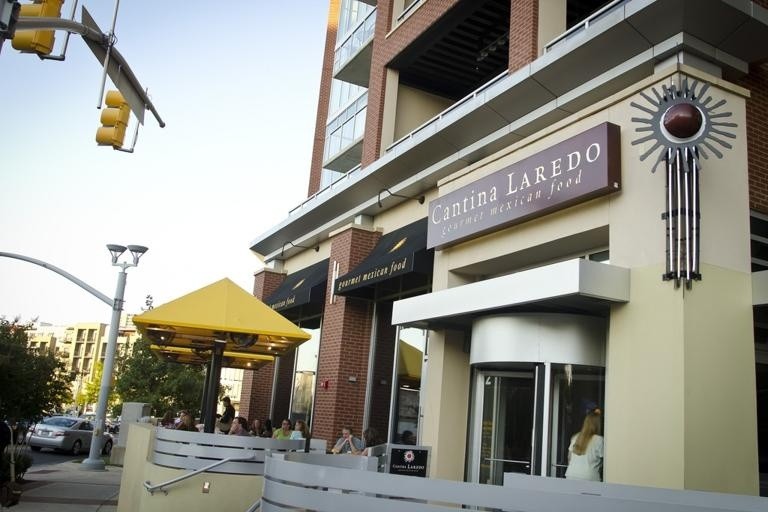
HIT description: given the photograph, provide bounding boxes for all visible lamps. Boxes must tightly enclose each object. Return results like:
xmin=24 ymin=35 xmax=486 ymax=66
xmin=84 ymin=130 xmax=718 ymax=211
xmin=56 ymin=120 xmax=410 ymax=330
xmin=377 ymin=187 xmax=426 ymax=208
xmin=281 ymin=241 xmax=320 ymax=257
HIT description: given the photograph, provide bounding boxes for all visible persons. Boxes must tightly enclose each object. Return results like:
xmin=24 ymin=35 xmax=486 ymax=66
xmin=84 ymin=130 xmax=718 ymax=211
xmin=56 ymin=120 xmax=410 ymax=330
xmin=562 ymin=411 xmax=604 ymax=479
xmin=159 ymin=395 xmax=311 ymax=453
xmin=330 ymin=426 xmax=363 ymax=452
xmin=399 ymin=431 xmax=414 ymax=444
xmin=356 ymin=427 xmax=385 ymax=456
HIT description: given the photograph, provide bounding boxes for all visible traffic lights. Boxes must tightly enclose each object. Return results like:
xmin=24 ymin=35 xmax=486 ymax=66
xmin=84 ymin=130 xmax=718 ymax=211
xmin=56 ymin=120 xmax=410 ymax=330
xmin=12 ymin=0 xmax=64 ymax=54
xmin=96 ymin=90 xmax=132 ymax=145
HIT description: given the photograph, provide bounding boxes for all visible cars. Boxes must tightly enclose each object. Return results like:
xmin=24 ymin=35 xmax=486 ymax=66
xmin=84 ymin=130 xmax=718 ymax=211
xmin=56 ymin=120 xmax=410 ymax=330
xmin=3 ymin=414 xmax=120 ymax=456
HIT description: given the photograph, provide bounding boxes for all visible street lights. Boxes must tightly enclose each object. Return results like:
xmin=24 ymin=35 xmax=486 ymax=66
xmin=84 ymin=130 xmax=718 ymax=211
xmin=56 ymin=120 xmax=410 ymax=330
xmin=0 ymin=245 xmax=148 ymax=470
